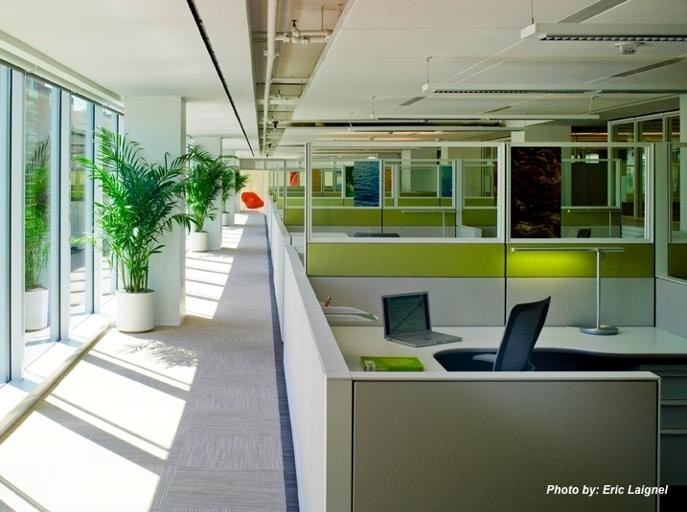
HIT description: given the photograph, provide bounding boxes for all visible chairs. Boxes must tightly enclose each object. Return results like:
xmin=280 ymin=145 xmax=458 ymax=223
xmin=471 ymin=296 xmax=552 ymax=372
xmin=578 ymin=228 xmax=592 ymax=237
xmin=241 ymin=193 xmax=263 ymax=209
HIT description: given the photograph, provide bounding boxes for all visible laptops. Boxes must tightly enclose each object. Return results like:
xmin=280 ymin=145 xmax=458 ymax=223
xmin=381 ymin=291 xmax=462 ymax=349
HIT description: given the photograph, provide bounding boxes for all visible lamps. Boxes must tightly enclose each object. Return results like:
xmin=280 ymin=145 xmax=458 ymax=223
xmin=512 ymin=246 xmax=625 ymax=337
xmin=520 ymin=1 xmax=687 ymax=45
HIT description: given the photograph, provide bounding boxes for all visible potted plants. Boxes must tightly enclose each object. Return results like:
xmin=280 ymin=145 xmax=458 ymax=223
xmin=76 ymin=128 xmax=233 ymax=333
xmin=25 ymin=135 xmax=49 ymax=332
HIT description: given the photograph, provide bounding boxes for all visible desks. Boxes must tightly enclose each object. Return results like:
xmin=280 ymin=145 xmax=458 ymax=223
xmin=291 ymin=233 xmax=350 ymax=254
xmin=328 ymin=325 xmax=686 ymax=488
